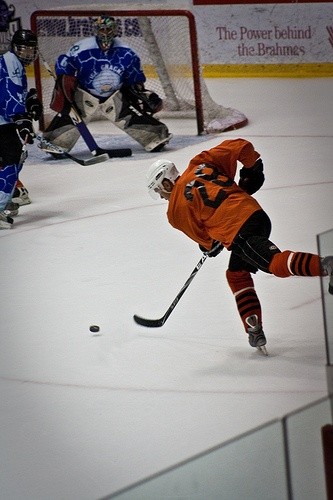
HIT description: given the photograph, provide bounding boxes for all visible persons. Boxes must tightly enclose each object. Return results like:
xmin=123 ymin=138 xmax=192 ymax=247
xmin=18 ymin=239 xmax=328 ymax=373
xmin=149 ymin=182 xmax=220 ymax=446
xmin=36 ymin=16 xmax=174 ymax=160
xmin=0 ymin=0 xmax=41 ymax=228
xmin=143 ymin=137 xmax=333 ymax=355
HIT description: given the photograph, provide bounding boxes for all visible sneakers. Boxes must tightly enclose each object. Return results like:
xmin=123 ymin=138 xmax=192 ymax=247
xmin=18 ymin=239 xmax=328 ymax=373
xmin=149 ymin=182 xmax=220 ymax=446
xmin=318 ymin=256 xmax=333 ymax=295
xmin=0 ymin=211 xmax=13 ymax=229
xmin=245 ymin=314 xmax=268 ymax=356
xmin=10 ymin=180 xmax=32 ymax=206
xmin=4 ymin=200 xmax=19 ymax=216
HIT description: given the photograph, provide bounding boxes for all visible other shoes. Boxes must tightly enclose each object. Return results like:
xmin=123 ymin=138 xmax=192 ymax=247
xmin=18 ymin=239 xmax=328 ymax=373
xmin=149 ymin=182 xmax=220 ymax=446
xmin=37 ymin=136 xmax=70 ymax=159
xmin=145 ymin=128 xmax=173 ymax=152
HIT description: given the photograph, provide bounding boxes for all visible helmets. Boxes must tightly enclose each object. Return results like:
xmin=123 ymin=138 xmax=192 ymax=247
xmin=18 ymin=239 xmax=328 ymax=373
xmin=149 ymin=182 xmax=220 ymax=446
xmin=145 ymin=159 xmax=180 ymax=193
xmin=10 ymin=29 xmax=38 ymax=46
xmin=96 ymin=28 xmax=114 ymax=42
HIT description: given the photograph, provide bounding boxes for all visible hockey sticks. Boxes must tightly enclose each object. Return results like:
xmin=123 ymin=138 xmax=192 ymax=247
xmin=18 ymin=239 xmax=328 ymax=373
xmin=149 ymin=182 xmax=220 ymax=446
xmin=37 ymin=50 xmax=133 ymax=160
xmin=131 ymin=254 xmax=209 ymax=328
xmin=29 ymin=131 xmax=110 ymax=167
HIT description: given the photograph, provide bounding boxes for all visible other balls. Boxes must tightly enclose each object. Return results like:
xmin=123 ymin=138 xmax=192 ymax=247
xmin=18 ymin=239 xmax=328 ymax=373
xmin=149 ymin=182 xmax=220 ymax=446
xmin=88 ymin=325 xmax=101 ymax=333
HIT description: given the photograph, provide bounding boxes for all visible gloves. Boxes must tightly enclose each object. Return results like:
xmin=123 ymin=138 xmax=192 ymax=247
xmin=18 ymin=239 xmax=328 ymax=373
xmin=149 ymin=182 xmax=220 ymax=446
xmin=138 ymin=90 xmax=163 ymax=114
xmin=199 ymin=240 xmax=225 ymax=258
xmin=239 ymin=159 xmax=265 ymax=195
xmin=11 ymin=112 xmax=37 ymax=145
xmin=25 ymin=88 xmax=43 ymax=121
